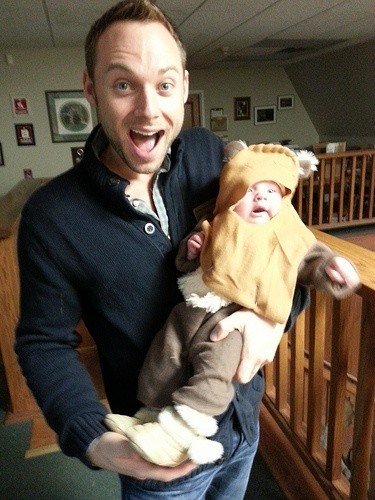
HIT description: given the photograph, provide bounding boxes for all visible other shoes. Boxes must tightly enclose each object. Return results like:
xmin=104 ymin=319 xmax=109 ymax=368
xmin=104 ymin=404 xmax=224 ymax=468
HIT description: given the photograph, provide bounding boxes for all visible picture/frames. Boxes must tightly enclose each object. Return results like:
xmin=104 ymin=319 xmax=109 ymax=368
xmin=45 ymin=89 xmax=99 ymax=143
xmin=183 ymin=89 xmax=206 ymax=129
xmin=234 ymin=96 xmax=251 ymax=121
xmin=15 ymin=123 xmax=36 ymax=145
xmin=277 ymin=94 xmax=294 ymax=111
xmin=254 ymin=105 xmax=277 ymax=126
xmin=11 ymin=96 xmax=31 ymax=118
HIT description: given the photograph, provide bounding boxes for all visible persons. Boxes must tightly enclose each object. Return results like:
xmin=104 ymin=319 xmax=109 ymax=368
xmin=12 ymin=0 xmax=308 ymax=500
xmin=105 ymin=139 xmax=360 ymax=468
